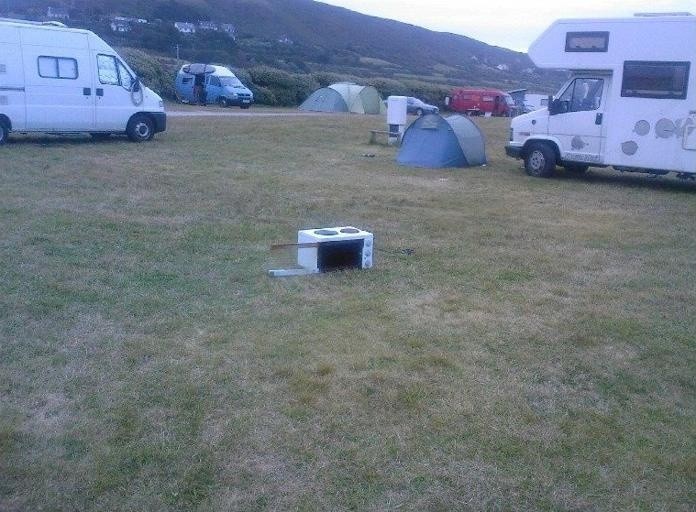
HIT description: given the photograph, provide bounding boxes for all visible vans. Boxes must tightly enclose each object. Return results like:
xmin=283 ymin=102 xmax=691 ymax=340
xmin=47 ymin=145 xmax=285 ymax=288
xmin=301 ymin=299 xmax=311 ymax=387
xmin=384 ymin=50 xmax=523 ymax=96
xmin=504 ymin=12 xmax=696 ymax=181
xmin=452 ymin=89 xmax=516 ymax=117
xmin=0 ymin=18 xmax=166 ymax=146
xmin=174 ymin=64 xmax=254 ymax=109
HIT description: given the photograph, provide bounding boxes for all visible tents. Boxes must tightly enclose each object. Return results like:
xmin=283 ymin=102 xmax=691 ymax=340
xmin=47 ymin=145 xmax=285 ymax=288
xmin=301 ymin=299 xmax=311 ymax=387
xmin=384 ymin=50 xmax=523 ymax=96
xmin=295 ymin=81 xmax=388 ymax=114
xmin=394 ymin=112 xmax=487 ymax=169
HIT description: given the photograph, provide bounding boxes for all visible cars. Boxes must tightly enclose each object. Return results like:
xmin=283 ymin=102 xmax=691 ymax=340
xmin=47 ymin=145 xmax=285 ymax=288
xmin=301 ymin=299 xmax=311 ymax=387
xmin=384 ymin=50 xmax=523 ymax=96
xmin=407 ymin=99 xmax=439 ymax=116
